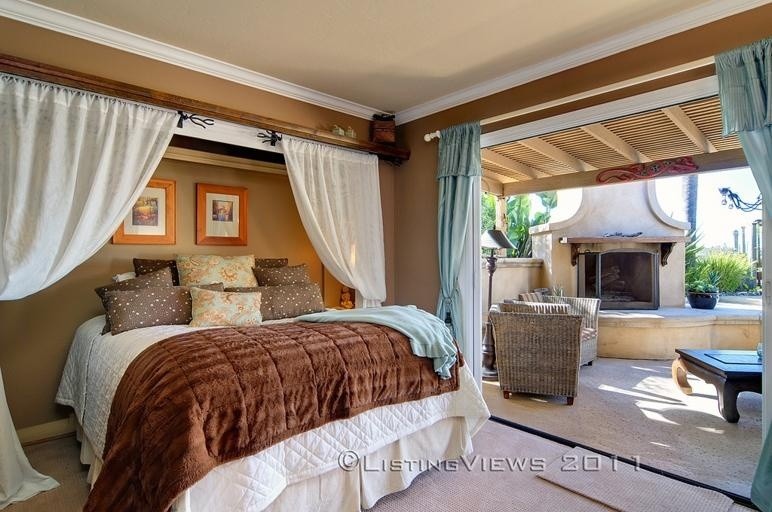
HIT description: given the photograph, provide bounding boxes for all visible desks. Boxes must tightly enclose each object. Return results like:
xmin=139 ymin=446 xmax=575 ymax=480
xmin=672 ymin=349 xmax=762 ymax=423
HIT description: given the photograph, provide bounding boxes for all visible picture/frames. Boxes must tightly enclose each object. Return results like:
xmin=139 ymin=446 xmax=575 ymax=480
xmin=111 ymin=178 xmax=177 ymax=246
xmin=196 ymin=182 xmax=248 ymax=247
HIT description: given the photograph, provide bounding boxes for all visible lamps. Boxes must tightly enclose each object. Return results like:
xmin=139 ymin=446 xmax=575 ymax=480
xmin=481 ymin=224 xmax=517 ymax=382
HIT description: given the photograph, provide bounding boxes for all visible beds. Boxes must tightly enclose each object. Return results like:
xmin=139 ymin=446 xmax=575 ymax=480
xmin=54 ymin=303 xmax=491 ymax=512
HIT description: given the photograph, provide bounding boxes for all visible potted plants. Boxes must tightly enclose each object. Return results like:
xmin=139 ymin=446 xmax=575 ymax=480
xmin=685 ymin=281 xmax=718 ymax=309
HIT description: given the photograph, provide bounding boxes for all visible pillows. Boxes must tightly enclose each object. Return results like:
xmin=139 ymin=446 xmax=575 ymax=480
xmin=94 ymin=254 xmax=325 ymax=336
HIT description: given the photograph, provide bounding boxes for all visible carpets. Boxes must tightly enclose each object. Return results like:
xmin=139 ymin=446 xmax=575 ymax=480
xmin=535 ymin=445 xmax=735 ymax=512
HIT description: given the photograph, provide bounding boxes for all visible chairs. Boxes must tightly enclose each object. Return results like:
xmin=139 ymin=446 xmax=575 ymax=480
xmin=489 ymin=293 xmax=602 ymax=406
